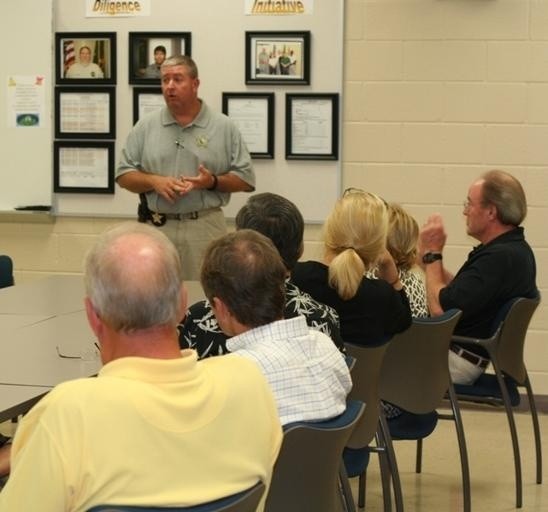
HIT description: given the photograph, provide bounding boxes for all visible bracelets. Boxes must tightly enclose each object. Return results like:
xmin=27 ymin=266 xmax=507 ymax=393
xmin=208 ymin=173 xmax=218 ymax=192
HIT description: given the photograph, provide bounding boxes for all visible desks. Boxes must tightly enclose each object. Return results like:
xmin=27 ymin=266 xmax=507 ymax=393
xmin=0 ymin=382 xmax=54 ymax=420
xmin=1 ymin=278 xmax=208 ymax=386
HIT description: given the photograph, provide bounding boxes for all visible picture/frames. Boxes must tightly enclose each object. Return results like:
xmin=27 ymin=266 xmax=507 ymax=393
xmin=127 ymin=30 xmax=193 ymax=85
xmin=284 ymin=91 xmax=339 ymax=161
xmin=52 ymin=142 xmax=116 ymax=195
xmin=55 ymin=31 xmax=117 ymax=84
xmin=222 ymin=91 xmax=276 ymax=160
xmin=132 ymin=87 xmax=169 ymax=128
xmin=243 ymin=29 xmax=311 ymax=86
xmin=54 ymin=87 xmax=117 ymax=139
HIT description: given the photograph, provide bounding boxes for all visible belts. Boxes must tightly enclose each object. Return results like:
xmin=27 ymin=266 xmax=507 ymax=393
xmin=152 ymin=207 xmax=222 ymax=221
xmin=450 ymin=345 xmax=491 ymax=369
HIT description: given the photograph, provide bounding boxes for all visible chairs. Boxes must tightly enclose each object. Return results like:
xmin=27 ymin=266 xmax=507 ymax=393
xmin=416 ymin=287 xmax=542 ymax=509
xmin=380 ymin=308 xmax=471 ymax=512
xmin=344 ymin=335 xmax=394 ymax=512
xmin=86 ymin=483 xmax=266 ymax=512
xmin=343 ymin=353 xmax=370 ymax=512
xmin=1 ymin=254 xmax=28 ymax=423
xmin=273 ymin=402 xmax=367 ymax=512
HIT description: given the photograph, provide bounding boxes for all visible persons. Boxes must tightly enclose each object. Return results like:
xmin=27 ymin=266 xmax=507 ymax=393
xmin=363 ymin=202 xmax=431 ymax=421
xmin=279 ymin=187 xmax=410 ymax=348
xmin=259 ymin=48 xmax=297 ymax=76
xmin=0 ymin=220 xmax=285 ymax=512
xmin=115 ymin=55 xmax=254 ymax=280
xmin=145 ymin=47 xmax=168 ymax=78
xmin=176 ymin=192 xmax=347 ymax=362
xmin=420 ymin=170 xmax=538 ymax=387
xmin=190 ymin=229 xmax=355 ymax=426
xmin=65 ymin=46 xmax=103 ymax=80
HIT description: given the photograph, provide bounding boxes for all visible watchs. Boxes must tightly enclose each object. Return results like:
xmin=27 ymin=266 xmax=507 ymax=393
xmin=420 ymin=253 xmax=443 ymax=264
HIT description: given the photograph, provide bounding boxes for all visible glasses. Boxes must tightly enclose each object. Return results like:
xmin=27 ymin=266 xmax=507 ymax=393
xmin=465 ymin=200 xmax=490 ymax=209
xmin=58 ymin=343 xmax=101 ymax=362
xmin=341 ymin=188 xmax=388 ymax=209
